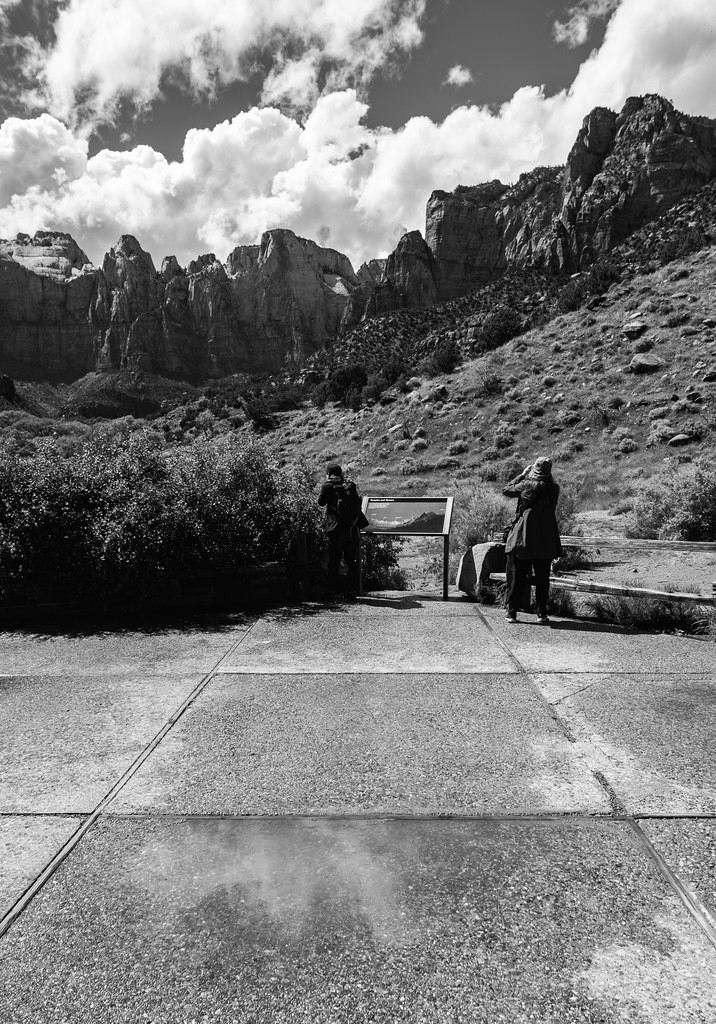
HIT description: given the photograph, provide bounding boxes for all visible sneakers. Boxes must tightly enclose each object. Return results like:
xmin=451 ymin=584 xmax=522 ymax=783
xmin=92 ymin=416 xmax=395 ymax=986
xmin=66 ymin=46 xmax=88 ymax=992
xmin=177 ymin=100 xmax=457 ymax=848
xmin=537 ymin=613 xmax=549 ymax=622
xmin=505 ymin=612 xmax=517 ymax=623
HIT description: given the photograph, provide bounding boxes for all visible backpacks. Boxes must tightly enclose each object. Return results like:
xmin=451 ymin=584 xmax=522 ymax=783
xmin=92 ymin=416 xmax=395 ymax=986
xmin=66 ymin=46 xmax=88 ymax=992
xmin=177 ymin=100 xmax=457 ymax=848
xmin=327 ymin=481 xmax=360 ymax=521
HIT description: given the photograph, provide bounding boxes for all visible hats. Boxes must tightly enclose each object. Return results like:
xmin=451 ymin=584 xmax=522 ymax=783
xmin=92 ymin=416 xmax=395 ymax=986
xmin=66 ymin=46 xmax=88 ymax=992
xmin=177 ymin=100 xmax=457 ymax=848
xmin=326 ymin=463 xmax=342 ymax=476
xmin=529 ymin=457 xmax=553 ymax=480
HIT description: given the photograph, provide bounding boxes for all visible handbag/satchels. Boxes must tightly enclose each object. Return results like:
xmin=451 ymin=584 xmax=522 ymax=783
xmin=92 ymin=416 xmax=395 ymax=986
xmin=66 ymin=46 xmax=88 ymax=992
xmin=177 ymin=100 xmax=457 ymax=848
xmin=502 ymin=518 xmax=515 ymax=543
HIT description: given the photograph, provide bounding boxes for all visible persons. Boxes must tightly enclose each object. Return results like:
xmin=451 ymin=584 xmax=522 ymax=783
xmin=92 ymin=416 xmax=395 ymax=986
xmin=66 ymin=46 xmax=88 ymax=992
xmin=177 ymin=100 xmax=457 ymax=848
xmin=502 ymin=456 xmax=564 ymax=623
xmin=318 ymin=464 xmax=369 ymax=603
